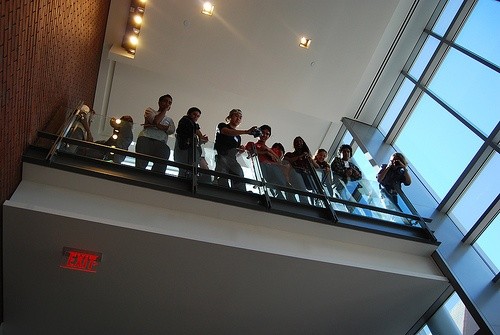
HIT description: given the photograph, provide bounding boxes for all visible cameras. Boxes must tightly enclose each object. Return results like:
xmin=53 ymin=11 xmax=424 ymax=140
xmin=252 ymin=128 xmax=263 ymax=139
xmin=389 ymin=159 xmax=399 ymax=168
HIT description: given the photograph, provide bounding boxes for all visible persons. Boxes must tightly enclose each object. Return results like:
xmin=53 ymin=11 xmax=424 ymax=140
xmin=85 ymin=116 xmax=134 ymax=163
xmin=331 ymin=144 xmax=363 ymax=214
xmin=284 ymin=136 xmax=319 ymax=206
xmin=245 ymin=125 xmax=297 ymax=202
xmin=67 ymin=109 xmax=96 ymax=153
xmin=174 ymin=106 xmax=211 ymax=182
xmin=376 ymin=152 xmax=411 ymax=203
xmin=313 ymin=149 xmax=332 ymax=207
xmin=213 ymin=108 xmax=257 ymax=190
xmin=135 ymin=95 xmax=175 ymax=173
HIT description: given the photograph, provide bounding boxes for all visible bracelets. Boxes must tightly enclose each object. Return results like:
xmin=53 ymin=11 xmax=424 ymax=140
xmin=404 ymin=168 xmax=408 ymax=172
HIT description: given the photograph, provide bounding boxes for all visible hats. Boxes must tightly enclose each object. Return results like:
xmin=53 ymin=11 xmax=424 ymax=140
xmin=225 ymin=109 xmax=242 ymax=122
